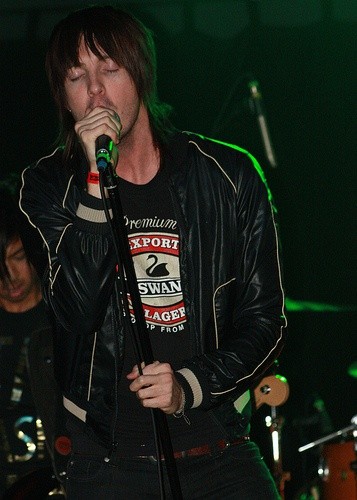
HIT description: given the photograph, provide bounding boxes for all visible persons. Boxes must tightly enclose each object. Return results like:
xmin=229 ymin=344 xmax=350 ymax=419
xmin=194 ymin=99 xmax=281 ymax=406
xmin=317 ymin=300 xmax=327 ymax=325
xmin=0 ymin=214 xmax=66 ymax=500
xmin=15 ymin=4 xmax=289 ymax=500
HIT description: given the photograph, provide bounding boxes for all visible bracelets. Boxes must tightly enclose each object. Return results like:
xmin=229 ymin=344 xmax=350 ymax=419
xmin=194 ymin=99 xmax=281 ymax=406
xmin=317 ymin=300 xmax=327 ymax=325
xmin=84 ymin=170 xmax=103 ymax=185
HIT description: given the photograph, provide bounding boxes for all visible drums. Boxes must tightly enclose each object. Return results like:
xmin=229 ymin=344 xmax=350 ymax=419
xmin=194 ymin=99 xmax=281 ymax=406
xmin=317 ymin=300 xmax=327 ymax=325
xmin=320 ymin=429 xmax=357 ymax=499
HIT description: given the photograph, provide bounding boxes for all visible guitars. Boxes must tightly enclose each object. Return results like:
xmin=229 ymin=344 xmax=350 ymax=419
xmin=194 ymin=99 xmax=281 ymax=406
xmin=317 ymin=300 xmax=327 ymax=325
xmin=253 ymin=373 xmax=291 ymax=412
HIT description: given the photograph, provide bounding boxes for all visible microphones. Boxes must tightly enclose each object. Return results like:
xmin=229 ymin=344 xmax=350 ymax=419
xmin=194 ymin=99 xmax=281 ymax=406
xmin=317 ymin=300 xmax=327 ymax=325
xmin=94 ymin=112 xmax=119 ymax=171
xmin=246 ymin=80 xmax=277 ymax=169
